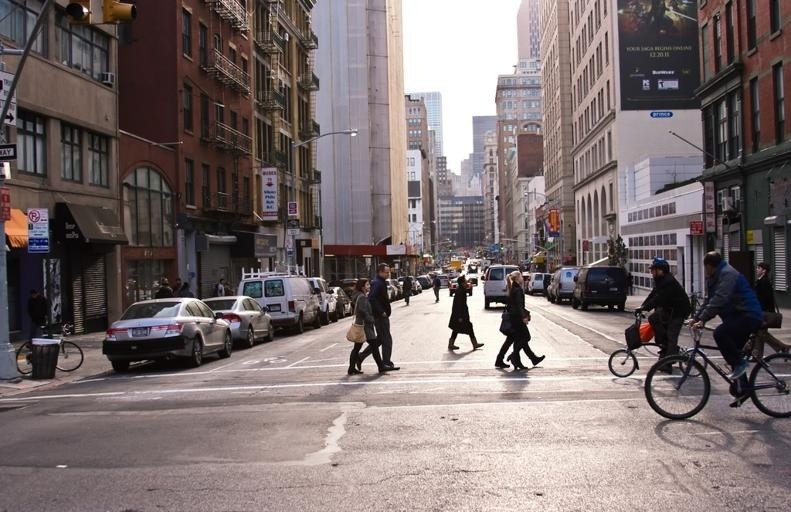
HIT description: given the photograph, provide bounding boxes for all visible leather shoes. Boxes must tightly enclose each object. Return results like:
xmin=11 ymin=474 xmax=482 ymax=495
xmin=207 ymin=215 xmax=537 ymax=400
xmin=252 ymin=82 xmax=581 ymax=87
xmin=356 ymin=353 xmax=361 ymax=370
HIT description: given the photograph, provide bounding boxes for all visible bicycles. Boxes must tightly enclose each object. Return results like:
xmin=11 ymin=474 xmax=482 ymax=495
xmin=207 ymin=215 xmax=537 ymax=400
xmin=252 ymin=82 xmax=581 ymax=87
xmin=645 ymin=322 xmax=791 ymax=420
xmin=17 ymin=323 xmax=83 ymax=375
xmin=608 ymin=310 xmax=708 ymax=376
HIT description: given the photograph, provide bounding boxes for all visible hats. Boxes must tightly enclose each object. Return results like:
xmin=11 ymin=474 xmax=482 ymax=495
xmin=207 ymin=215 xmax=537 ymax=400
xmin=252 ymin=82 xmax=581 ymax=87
xmin=647 ymin=256 xmax=669 ymax=269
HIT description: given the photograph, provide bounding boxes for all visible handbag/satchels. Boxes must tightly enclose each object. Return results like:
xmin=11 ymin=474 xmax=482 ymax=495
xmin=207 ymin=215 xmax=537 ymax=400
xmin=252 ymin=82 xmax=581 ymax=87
xmin=761 ymin=311 xmax=782 ymax=328
xmin=346 ymin=324 xmax=367 ymax=343
xmin=500 ymin=312 xmax=514 ymax=336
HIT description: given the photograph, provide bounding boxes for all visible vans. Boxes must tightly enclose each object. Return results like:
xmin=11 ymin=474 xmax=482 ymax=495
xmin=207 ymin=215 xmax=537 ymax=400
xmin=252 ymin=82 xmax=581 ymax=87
xmin=237 ymin=275 xmax=323 ymax=333
xmin=552 ymin=268 xmax=577 ymax=303
xmin=485 ymin=265 xmax=523 ymax=309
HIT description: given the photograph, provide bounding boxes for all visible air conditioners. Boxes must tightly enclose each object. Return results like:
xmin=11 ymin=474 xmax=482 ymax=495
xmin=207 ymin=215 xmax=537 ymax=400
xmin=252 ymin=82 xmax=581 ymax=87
xmin=102 ymin=72 xmax=115 ymax=85
xmin=267 ymin=69 xmax=276 ymax=80
xmin=721 ymin=196 xmax=735 ymax=212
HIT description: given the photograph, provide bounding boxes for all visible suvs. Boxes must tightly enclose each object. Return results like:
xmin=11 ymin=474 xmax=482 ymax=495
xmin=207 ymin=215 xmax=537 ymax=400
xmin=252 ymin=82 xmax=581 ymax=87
xmin=307 ymin=278 xmax=337 ymax=324
xmin=570 ymin=266 xmax=626 ymax=310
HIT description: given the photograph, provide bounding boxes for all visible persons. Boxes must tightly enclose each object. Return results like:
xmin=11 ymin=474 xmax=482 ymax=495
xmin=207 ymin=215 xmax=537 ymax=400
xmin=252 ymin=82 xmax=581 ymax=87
xmin=154 ymin=271 xmax=236 ymax=305
xmin=507 ymin=271 xmax=530 ymax=371
xmin=447 ymin=275 xmax=485 ymax=350
xmin=495 ymin=285 xmax=546 ymax=369
xmin=746 ymin=262 xmax=776 ymax=361
xmin=356 ymin=261 xmax=402 ymax=371
xmin=403 ymin=275 xmax=413 ymax=305
xmin=634 ymin=257 xmax=692 ymax=375
xmin=686 ymin=250 xmax=768 ymax=409
xmin=347 ymin=277 xmax=394 ymax=375
xmin=432 ymin=274 xmax=441 ymax=302
xmin=627 ymin=0 xmax=688 ymax=34
xmin=25 ymin=285 xmax=53 ymax=343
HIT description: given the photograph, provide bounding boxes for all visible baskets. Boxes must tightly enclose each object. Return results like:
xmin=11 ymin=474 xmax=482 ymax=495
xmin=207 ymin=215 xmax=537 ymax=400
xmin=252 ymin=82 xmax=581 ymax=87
xmin=625 ymin=323 xmax=642 ymax=350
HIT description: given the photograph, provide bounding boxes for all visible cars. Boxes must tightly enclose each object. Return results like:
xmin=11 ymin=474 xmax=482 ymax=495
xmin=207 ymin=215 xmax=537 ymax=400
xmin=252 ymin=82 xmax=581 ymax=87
xmin=329 ymin=271 xmax=448 ymax=318
xmin=468 ymin=264 xmax=550 ymax=297
xmin=449 ymin=279 xmax=473 ymax=297
xmin=449 ymin=260 xmax=465 ymax=277
xmin=104 ymin=299 xmax=232 ymax=373
xmin=202 ymin=296 xmax=274 ymax=347
xmin=547 ymin=275 xmax=552 ymax=300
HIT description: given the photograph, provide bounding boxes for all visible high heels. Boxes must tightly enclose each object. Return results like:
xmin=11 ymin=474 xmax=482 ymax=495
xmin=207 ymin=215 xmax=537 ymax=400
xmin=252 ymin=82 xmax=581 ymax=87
xmin=514 ymin=361 xmax=528 ymax=370
xmin=507 ymin=352 xmax=518 ymax=367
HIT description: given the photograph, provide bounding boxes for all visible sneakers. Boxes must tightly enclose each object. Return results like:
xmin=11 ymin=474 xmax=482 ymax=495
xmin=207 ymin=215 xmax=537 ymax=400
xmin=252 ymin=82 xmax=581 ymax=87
xmin=728 ymin=360 xmax=749 ymax=379
xmin=729 ymin=392 xmax=749 ymax=407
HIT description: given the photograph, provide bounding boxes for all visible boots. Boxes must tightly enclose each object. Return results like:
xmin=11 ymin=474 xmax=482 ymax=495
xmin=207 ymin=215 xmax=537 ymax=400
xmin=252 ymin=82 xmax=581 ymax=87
xmin=372 ymin=348 xmax=392 ymax=371
xmin=448 ymin=338 xmax=460 ymax=349
xmin=495 ymin=357 xmax=510 ymax=368
xmin=348 ymin=349 xmax=363 ymax=374
xmin=529 ymin=354 xmax=545 ymax=365
xmin=471 ymin=337 xmax=484 ymax=349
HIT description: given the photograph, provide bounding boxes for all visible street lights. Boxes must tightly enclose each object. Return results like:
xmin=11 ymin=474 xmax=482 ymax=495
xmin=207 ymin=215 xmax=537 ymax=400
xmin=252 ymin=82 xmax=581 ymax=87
xmin=288 ymin=127 xmax=358 ymax=200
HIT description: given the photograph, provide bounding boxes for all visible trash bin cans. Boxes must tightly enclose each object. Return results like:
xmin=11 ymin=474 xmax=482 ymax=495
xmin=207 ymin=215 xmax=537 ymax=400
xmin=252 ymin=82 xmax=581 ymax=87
xmin=32 ymin=338 xmax=61 ymax=378
